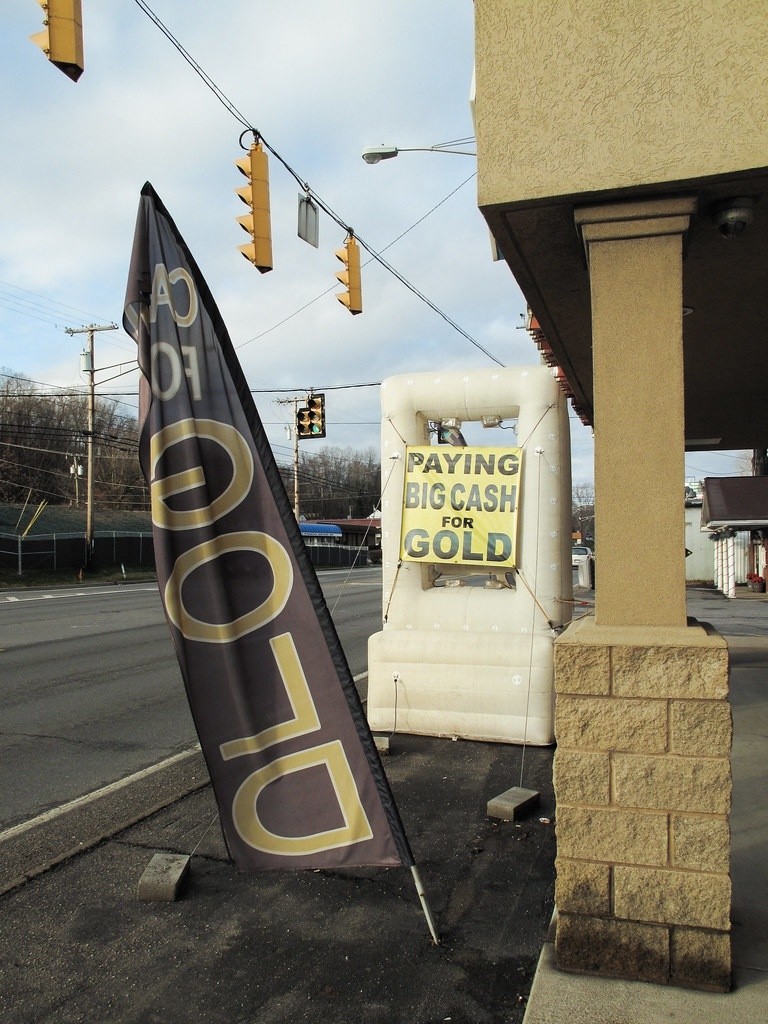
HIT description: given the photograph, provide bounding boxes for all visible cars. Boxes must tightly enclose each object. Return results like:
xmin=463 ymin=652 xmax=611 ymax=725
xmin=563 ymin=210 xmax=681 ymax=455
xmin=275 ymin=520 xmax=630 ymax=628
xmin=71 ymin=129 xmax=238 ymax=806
xmin=570 ymin=547 xmax=593 ymax=569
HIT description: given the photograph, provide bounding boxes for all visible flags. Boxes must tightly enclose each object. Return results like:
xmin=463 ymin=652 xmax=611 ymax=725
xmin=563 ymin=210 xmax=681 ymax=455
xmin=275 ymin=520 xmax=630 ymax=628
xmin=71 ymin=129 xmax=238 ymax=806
xmin=122 ymin=188 xmax=402 ymax=870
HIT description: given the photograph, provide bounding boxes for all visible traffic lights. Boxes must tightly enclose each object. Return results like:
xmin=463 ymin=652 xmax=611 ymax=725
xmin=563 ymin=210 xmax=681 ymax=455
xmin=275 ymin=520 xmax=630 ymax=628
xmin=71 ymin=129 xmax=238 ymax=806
xmin=298 ymin=408 xmax=310 ymax=435
xmin=308 ymin=394 xmax=325 ymax=435
